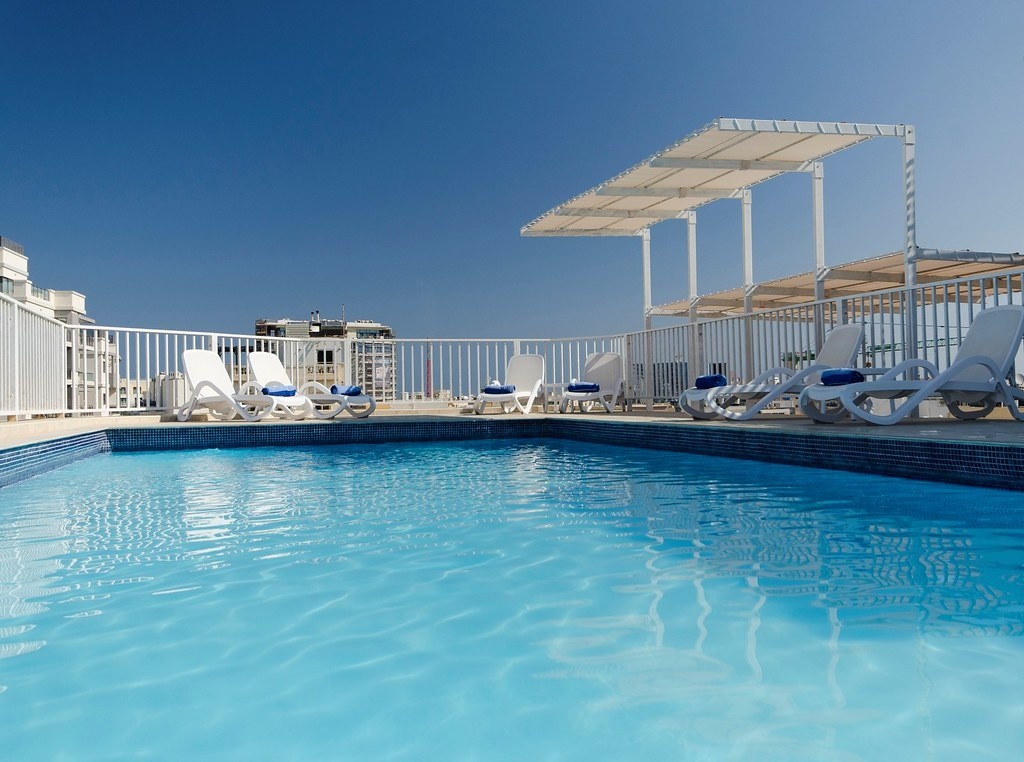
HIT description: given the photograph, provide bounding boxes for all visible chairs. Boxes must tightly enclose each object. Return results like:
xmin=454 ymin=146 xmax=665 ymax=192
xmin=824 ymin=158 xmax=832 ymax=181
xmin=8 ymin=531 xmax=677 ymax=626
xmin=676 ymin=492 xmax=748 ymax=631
xmin=473 ymin=354 xmax=549 ymax=415
xmin=176 ymin=349 xmax=314 ymax=422
xmin=241 ymin=351 xmax=377 ymax=420
xmin=797 ymin=305 xmax=1024 ymax=426
xmin=679 ymin=323 xmax=873 ymax=421
xmin=558 ymin=352 xmax=626 ymax=413
